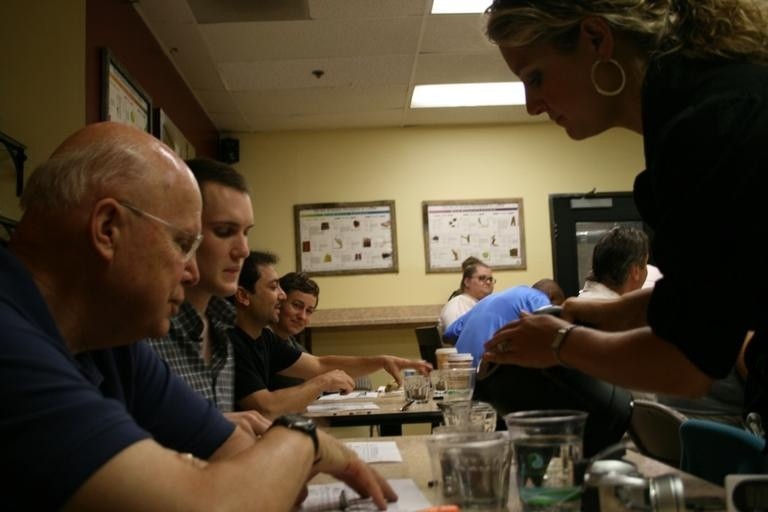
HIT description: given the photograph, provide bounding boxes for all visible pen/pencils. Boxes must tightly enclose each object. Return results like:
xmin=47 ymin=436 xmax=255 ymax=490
xmin=385 ymin=384 xmax=392 ymax=392
xmin=339 ymin=490 xmax=350 ymax=512
xmin=401 ymin=400 xmax=415 ymax=412
xmin=414 ymin=505 xmax=460 ymax=512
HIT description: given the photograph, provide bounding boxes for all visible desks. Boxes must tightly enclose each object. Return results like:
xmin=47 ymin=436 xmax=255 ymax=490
xmin=285 ymin=384 xmax=445 ymax=439
xmin=294 ymin=426 xmax=733 ymax=512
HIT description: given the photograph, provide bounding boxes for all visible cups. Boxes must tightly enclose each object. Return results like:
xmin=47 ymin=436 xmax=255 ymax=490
xmin=433 ymin=347 xmax=497 ymax=435
xmin=504 ymin=408 xmax=590 ymax=512
xmin=403 ymin=376 xmax=430 ymax=403
xmin=424 ymin=433 xmax=512 ymax=511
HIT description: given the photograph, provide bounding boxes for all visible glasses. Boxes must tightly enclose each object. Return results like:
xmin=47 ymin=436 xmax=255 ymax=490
xmin=116 ymin=201 xmax=203 ymax=262
xmin=472 ymin=276 xmax=496 ymax=285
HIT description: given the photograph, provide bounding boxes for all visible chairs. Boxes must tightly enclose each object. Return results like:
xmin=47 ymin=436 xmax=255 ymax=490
xmin=678 ymin=419 xmax=768 ymax=487
xmin=627 ymin=399 xmax=691 ymax=471
xmin=415 ymin=326 xmax=442 ymax=370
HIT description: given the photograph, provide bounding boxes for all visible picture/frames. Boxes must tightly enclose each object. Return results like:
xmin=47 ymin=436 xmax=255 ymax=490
xmin=157 ymin=106 xmax=196 ymax=161
xmin=99 ymin=45 xmax=153 ymax=135
xmin=292 ymin=199 xmax=399 ymax=276
xmin=422 ymin=197 xmax=528 ymax=273
